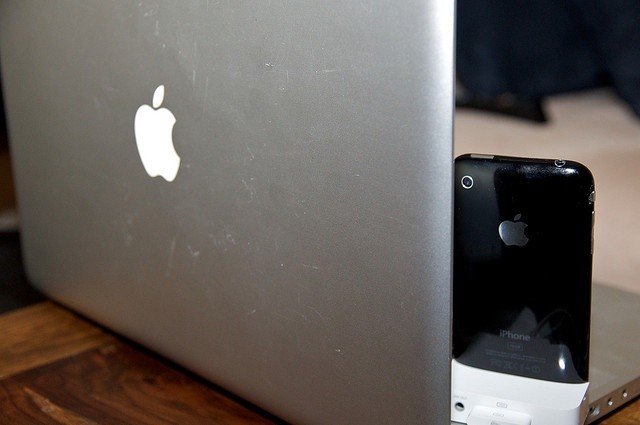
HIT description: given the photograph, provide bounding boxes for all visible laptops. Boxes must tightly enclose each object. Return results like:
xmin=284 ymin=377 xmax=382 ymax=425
xmin=2 ymin=0 xmax=640 ymax=424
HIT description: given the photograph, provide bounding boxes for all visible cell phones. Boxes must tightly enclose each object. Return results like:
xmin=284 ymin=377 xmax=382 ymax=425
xmin=452 ymin=153 xmax=596 ymax=384
